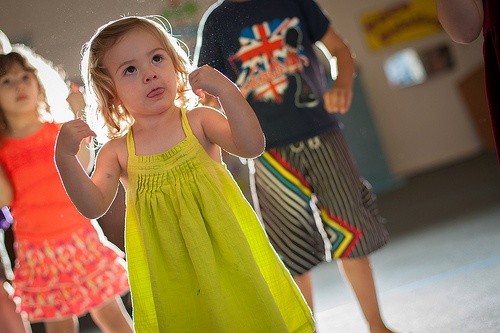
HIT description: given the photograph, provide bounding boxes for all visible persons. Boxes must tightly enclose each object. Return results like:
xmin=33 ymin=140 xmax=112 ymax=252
xmin=54 ymin=16 xmax=317 ymax=333
xmin=0 ymin=52 xmax=135 ymax=333
xmin=193 ymin=0 xmax=396 ymax=332
